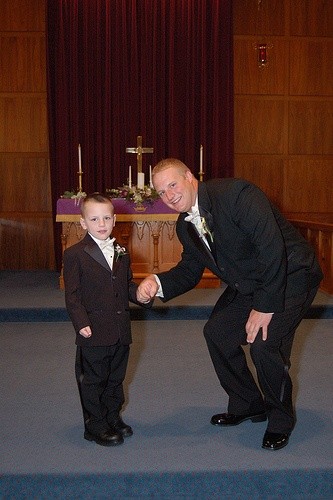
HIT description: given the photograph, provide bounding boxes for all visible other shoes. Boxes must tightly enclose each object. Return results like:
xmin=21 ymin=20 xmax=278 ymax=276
xmin=84 ymin=420 xmax=134 ymax=445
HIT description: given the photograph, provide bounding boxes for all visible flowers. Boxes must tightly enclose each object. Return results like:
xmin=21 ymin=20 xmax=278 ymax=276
xmin=114 ymin=244 xmax=125 ymax=263
xmin=195 ymin=216 xmax=214 ymax=243
xmin=62 ymin=185 xmax=160 ymax=209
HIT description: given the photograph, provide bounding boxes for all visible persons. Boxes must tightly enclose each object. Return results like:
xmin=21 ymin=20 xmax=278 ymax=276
xmin=137 ymin=159 xmax=325 ymax=449
xmin=63 ymin=192 xmax=152 ymax=445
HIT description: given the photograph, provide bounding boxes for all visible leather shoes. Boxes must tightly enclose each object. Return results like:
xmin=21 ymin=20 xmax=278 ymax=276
xmin=209 ymin=411 xmax=267 ymax=427
xmin=262 ymin=429 xmax=290 ymax=451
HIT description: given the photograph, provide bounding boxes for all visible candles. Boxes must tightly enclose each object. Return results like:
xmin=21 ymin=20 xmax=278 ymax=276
xmin=199 ymin=145 xmax=203 ymax=173
xmin=78 ymin=144 xmax=82 ymax=172
xmin=129 ymin=165 xmax=132 ymax=188
xmin=150 ymin=165 xmax=153 ymax=186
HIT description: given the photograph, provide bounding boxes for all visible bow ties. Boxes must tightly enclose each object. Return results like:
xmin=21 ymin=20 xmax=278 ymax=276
xmin=184 ymin=210 xmax=200 ymax=225
xmin=99 ymin=237 xmax=116 ymax=250
